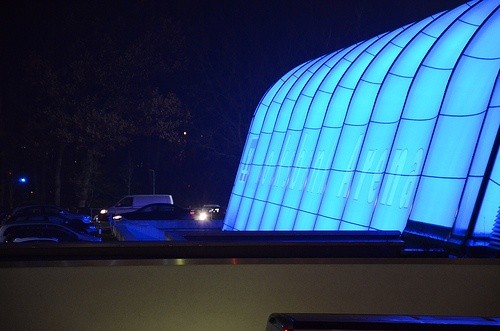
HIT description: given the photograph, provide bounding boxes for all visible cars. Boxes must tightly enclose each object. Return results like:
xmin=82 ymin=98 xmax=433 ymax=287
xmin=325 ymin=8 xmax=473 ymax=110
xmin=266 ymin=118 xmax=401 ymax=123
xmin=113 ymin=203 xmax=190 ymax=220
xmin=0 ymin=203 xmax=103 ymax=244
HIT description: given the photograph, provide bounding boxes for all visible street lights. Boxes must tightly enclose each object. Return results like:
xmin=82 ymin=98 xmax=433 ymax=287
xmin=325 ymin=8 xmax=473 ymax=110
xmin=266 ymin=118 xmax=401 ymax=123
xmin=149 ymin=168 xmax=156 ymax=195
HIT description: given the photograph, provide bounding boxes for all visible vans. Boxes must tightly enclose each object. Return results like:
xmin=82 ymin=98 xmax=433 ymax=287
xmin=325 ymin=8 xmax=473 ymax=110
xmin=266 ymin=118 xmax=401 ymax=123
xmin=105 ymin=194 xmax=174 ymax=224
